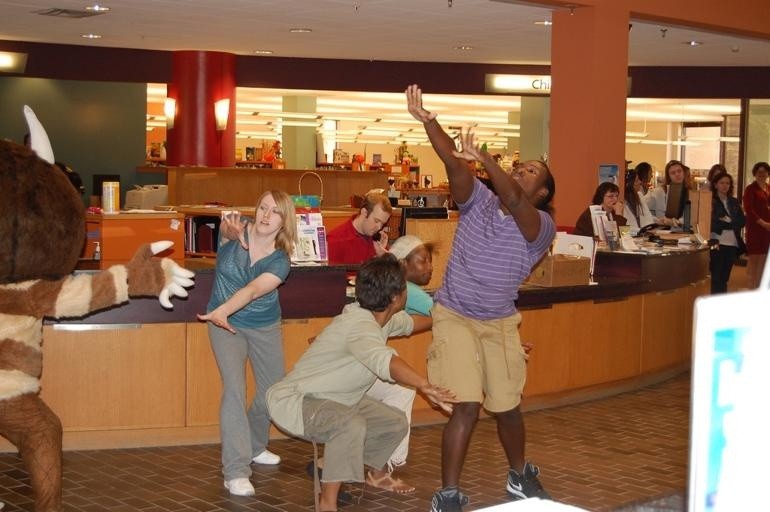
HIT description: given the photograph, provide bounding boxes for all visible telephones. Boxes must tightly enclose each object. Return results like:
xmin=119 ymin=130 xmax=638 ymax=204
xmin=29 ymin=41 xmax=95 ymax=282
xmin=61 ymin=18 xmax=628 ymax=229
xmin=372 ymin=224 xmax=388 ymax=243
xmin=639 ymin=223 xmax=671 ymax=233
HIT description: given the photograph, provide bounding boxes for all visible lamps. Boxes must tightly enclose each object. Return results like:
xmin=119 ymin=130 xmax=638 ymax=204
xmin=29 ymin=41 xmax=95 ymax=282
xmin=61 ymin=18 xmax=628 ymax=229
xmin=214 ymin=97 xmax=231 ymax=131
xmin=163 ymin=97 xmax=176 ymax=130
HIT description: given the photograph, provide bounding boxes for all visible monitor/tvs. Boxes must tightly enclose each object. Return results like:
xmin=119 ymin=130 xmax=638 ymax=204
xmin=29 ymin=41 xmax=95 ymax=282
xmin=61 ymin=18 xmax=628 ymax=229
xmin=665 ymin=182 xmax=694 ymax=234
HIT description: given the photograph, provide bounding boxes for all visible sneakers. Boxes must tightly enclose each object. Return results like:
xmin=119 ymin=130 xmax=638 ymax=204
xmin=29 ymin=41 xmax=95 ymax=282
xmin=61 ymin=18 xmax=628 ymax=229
xmin=252 ymin=450 xmax=280 ymax=465
xmin=431 ymin=486 xmax=468 ymax=512
xmin=307 ymin=461 xmax=353 ymax=505
xmin=506 ymin=460 xmax=553 ymax=501
xmin=224 ymin=478 xmax=255 ymax=496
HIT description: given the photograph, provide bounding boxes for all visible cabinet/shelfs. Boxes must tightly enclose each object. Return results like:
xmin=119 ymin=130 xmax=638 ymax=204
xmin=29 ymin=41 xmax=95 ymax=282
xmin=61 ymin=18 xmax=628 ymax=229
xmin=154 ymin=204 xmax=358 ymax=274
xmin=76 ymin=208 xmax=185 ymax=280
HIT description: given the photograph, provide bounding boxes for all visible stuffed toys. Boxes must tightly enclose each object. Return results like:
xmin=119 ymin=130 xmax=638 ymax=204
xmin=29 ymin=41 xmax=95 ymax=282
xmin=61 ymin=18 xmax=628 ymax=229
xmin=1 ymin=105 xmax=197 ymax=512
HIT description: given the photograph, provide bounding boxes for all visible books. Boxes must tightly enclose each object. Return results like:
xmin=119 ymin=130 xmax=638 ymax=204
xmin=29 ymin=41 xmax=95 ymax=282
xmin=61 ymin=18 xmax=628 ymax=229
xmin=182 ymin=214 xmax=197 ymax=254
xmin=286 ymin=193 xmax=330 ymax=262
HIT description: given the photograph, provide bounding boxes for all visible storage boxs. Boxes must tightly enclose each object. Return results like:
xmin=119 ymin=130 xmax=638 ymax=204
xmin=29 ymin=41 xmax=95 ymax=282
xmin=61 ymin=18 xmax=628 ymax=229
xmin=525 ymin=253 xmax=592 ymax=287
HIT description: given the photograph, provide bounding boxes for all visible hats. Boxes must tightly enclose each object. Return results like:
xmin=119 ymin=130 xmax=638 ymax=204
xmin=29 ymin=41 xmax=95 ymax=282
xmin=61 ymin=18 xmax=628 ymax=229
xmin=388 ymin=235 xmax=423 ymax=261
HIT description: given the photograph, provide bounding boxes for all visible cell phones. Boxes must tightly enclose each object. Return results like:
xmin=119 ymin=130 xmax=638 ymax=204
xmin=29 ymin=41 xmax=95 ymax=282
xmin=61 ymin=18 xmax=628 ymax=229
xmin=373 ymin=224 xmax=386 ymax=239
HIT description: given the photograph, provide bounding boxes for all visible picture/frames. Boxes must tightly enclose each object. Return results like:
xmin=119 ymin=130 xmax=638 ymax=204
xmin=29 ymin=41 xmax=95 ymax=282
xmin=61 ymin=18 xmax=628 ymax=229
xmin=421 ymin=174 xmax=433 ymax=189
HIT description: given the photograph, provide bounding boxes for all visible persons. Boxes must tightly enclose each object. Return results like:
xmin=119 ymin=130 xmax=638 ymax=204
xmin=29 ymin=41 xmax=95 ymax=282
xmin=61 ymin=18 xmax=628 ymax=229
xmin=323 ymin=196 xmax=394 ymax=267
xmin=578 ymin=150 xmax=694 ymax=245
xmin=705 ymin=165 xmax=730 ymax=185
xmin=196 ymin=188 xmax=298 ymax=498
xmin=744 ymin=164 xmax=769 ymax=289
xmin=707 ymin=175 xmax=746 ymax=294
xmin=404 ymin=83 xmax=553 ymax=511
xmin=381 ymin=232 xmax=534 ymax=361
xmin=263 ymin=253 xmax=460 ymax=512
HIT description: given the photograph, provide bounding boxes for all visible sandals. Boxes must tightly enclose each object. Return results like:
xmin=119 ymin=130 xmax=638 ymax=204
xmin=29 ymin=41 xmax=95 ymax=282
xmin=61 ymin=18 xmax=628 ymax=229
xmin=366 ymin=471 xmax=416 ymax=496
